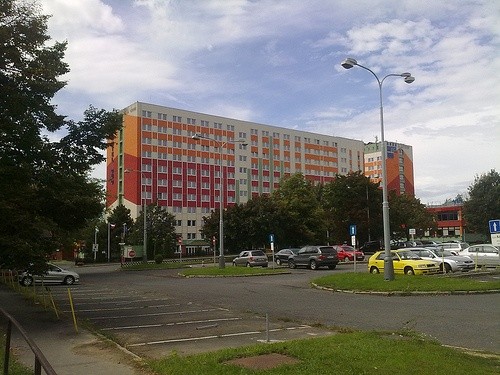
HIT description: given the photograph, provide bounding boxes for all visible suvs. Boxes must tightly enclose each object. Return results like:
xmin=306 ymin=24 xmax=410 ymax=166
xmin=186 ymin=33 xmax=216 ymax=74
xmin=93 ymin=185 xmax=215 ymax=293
xmin=288 ymin=246 xmax=339 ymax=271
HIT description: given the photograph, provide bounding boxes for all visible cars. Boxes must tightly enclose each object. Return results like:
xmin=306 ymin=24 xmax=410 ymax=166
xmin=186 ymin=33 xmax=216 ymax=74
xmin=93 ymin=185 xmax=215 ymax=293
xmin=232 ymin=250 xmax=268 ymax=268
xmin=330 ymin=245 xmax=365 ymax=262
xmin=390 ymin=239 xmax=469 ymax=257
xmin=274 ymin=249 xmax=301 ymax=266
xmin=367 ymin=249 xmax=440 ymax=276
xmin=396 ymin=248 xmax=475 ymax=273
xmin=458 ymin=244 xmax=500 ymax=268
xmin=15 ymin=262 xmax=80 ymax=287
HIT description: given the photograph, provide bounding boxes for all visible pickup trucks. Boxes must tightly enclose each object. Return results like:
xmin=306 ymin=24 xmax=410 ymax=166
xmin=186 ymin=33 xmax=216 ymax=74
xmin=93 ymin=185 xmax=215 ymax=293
xmin=359 ymin=240 xmax=385 ymax=255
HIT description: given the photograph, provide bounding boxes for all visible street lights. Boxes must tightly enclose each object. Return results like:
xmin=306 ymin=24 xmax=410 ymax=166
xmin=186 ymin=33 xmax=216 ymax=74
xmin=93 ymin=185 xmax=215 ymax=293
xmin=340 ymin=57 xmax=415 ymax=283
xmin=107 ymin=223 xmax=116 ymax=263
xmin=191 ymin=133 xmax=248 ymax=269
xmin=123 ymin=169 xmax=150 ymax=265
